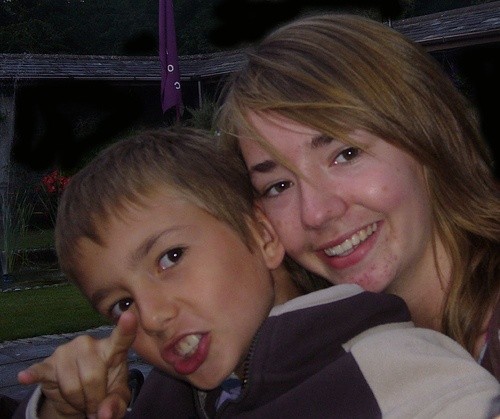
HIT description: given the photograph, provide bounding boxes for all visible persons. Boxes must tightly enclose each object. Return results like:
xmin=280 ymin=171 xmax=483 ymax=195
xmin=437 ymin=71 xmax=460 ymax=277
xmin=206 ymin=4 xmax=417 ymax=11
xmin=0 ymin=122 xmax=500 ymax=419
xmin=208 ymin=13 xmax=500 ymax=382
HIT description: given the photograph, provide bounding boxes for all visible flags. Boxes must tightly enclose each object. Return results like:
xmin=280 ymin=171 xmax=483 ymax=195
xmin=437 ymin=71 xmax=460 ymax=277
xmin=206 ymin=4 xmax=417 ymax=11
xmin=158 ymin=1 xmax=181 ymax=112
